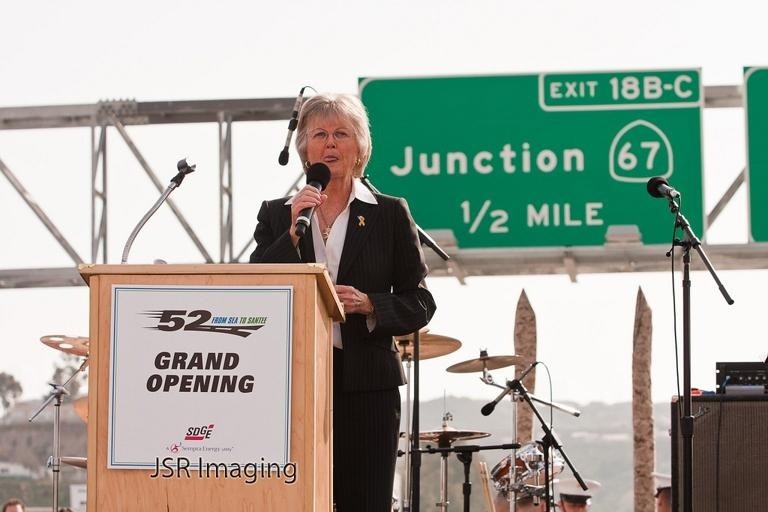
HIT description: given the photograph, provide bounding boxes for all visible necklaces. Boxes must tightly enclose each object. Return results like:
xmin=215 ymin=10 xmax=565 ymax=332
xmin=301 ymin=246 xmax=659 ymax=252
xmin=315 ymin=196 xmax=350 ymax=241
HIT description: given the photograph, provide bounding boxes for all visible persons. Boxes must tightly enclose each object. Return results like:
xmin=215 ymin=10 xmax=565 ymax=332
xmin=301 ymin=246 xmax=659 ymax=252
xmin=250 ymin=88 xmax=438 ymax=510
xmin=1 ymin=498 xmax=26 ymax=512
xmin=556 ymin=490 xmax=593 ymax=512
xmin=654 ymin=485 xmax=673 ymax=511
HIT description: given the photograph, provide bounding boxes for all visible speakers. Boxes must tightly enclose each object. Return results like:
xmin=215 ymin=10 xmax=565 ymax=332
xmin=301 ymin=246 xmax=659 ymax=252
xmin=670 ymin=393 xmax=768 ymax=512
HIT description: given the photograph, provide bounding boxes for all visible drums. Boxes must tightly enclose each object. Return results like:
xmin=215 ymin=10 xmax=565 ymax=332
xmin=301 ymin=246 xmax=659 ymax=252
xmin=492 ymin=440 xmax=565 ymax=503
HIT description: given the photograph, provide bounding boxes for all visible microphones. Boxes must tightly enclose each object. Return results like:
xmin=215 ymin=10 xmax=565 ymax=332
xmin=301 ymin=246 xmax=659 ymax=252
xmin=295 ymin=163 xmax=331 ymax=236
xmin=279 ymin=87 xmax=305 ymax=166
xmin=480 ymin=362 xmax=538 ymax=416
xmin=647 ymin=176 xmax=680 ymax=198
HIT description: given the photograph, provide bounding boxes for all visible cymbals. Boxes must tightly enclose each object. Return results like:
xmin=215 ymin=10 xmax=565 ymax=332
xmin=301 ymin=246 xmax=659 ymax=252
xmin=73 ymin=396 xmax=88 ymax=417
xmin=394 ymin=334 xmax=461 ymax=361
xmin=402 ymin=428 xmax=491 ymax=444
xmin=446 ymin=355 xmax=527 ymax=372
xmin=40 ymin=335 xmax=89 ymax=356
xmin=59 ymin=457 xmax=87 ymax=468
